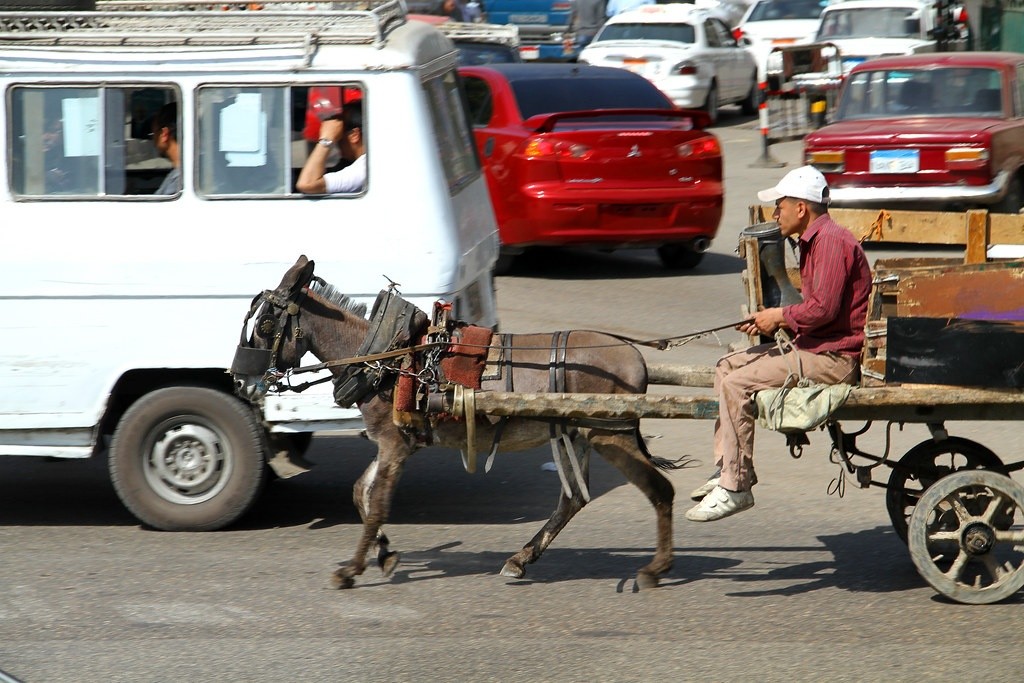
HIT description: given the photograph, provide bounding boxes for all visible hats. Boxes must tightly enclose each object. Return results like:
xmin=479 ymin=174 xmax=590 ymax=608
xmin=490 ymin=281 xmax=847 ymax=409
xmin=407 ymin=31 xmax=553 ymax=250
xmin=142 ymin=101 xmax=177 ymax=131
xmin=757 ymin=165 xmax=831 ymax=205
xmin=325 ymin=98 xmax=363 ymax=127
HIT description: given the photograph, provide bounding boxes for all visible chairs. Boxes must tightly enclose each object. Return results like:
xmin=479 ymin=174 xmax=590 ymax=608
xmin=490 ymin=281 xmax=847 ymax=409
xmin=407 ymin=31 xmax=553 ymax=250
xmin=893 ymin=80 xmax=924 ymax=112
xmin=972 ymin=88 xmax=999 ymax=110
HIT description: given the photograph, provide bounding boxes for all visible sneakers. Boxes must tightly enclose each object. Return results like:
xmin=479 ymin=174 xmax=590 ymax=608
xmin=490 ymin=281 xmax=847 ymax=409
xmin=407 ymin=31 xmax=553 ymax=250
xmin=689 ymin=466 xmax=758 ymax=500
xmin=684 ymin=486 xmax=755 ymax=523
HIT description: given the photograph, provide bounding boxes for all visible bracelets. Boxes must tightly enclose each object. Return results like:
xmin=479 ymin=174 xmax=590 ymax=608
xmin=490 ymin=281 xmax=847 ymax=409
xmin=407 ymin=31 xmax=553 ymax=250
xmin=319 ymin=139 xmax=334 ymax=147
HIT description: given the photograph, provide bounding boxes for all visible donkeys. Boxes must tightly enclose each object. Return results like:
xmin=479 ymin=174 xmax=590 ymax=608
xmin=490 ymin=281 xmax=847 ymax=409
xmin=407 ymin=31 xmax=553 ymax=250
xmin=229 ymin=252 xmax=703 ymax=597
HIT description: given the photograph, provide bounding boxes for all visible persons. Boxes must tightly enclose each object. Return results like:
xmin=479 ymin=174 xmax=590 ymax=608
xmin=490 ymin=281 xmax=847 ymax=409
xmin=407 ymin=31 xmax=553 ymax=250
xmin=152 ymin=103 xmax=180 ymax=195
xmin=685 ymin=165 xmax=872 ymax=523
xmin=24 ymin=109 xmax=83 ymax=195
xmin=429 ymin=0 xmax=464 ymax=22
xmin=296 ymin=98 xmax=366 ymax=194
xmin=885 ymin=81 xmax=921 ymax=111
